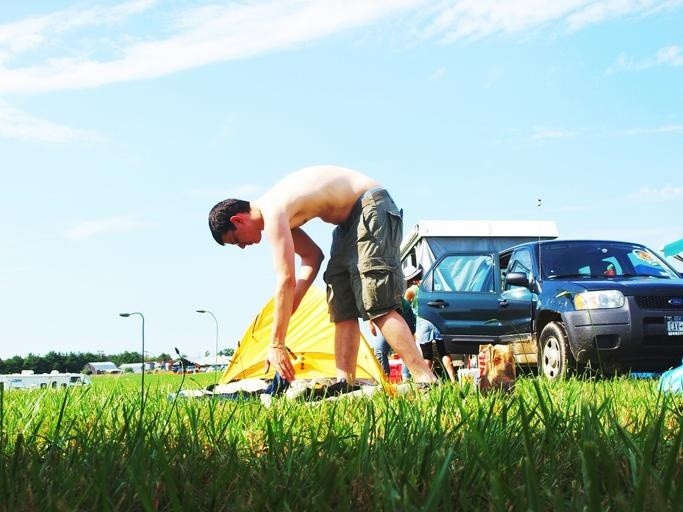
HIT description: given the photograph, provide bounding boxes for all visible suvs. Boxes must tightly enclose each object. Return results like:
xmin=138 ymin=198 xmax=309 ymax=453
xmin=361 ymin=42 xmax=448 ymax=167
xmin=413 ymin=238 xmax=682 ymax=381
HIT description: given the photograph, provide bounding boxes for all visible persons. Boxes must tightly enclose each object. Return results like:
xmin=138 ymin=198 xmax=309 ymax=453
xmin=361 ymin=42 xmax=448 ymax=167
xmin=195 ymin=363 xmax=200 ymax=373
xmin=367 ymin=318 xmax=411 ymax=383
xmin=414 ymin=315 xmax=456 ymax=383
xmin=404 ymin=274 xmax=422 ymax=312
xmin=209 ymin=163 xmax=441 ymax=391
xmin=145 ymin=358 xmax=173 ymax=372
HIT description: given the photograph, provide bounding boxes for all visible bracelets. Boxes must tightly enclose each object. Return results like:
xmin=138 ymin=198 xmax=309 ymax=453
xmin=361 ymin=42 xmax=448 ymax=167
xmin=270 ymin=344 xmax=287 ymax=350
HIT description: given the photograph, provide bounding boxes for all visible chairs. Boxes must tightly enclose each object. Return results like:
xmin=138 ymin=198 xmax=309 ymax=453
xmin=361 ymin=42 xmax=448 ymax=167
xmin=553 ymin=259 xmax=616 ymax=278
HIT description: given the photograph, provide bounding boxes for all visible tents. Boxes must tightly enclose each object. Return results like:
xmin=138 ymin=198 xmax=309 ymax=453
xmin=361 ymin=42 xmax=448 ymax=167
xmin=218 ymin=278 xmax=385 ymax=388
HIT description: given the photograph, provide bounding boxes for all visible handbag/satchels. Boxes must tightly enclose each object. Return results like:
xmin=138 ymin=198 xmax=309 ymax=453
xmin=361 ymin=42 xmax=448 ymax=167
xmin=478 ymin=343 xmax=516 ymax=399
